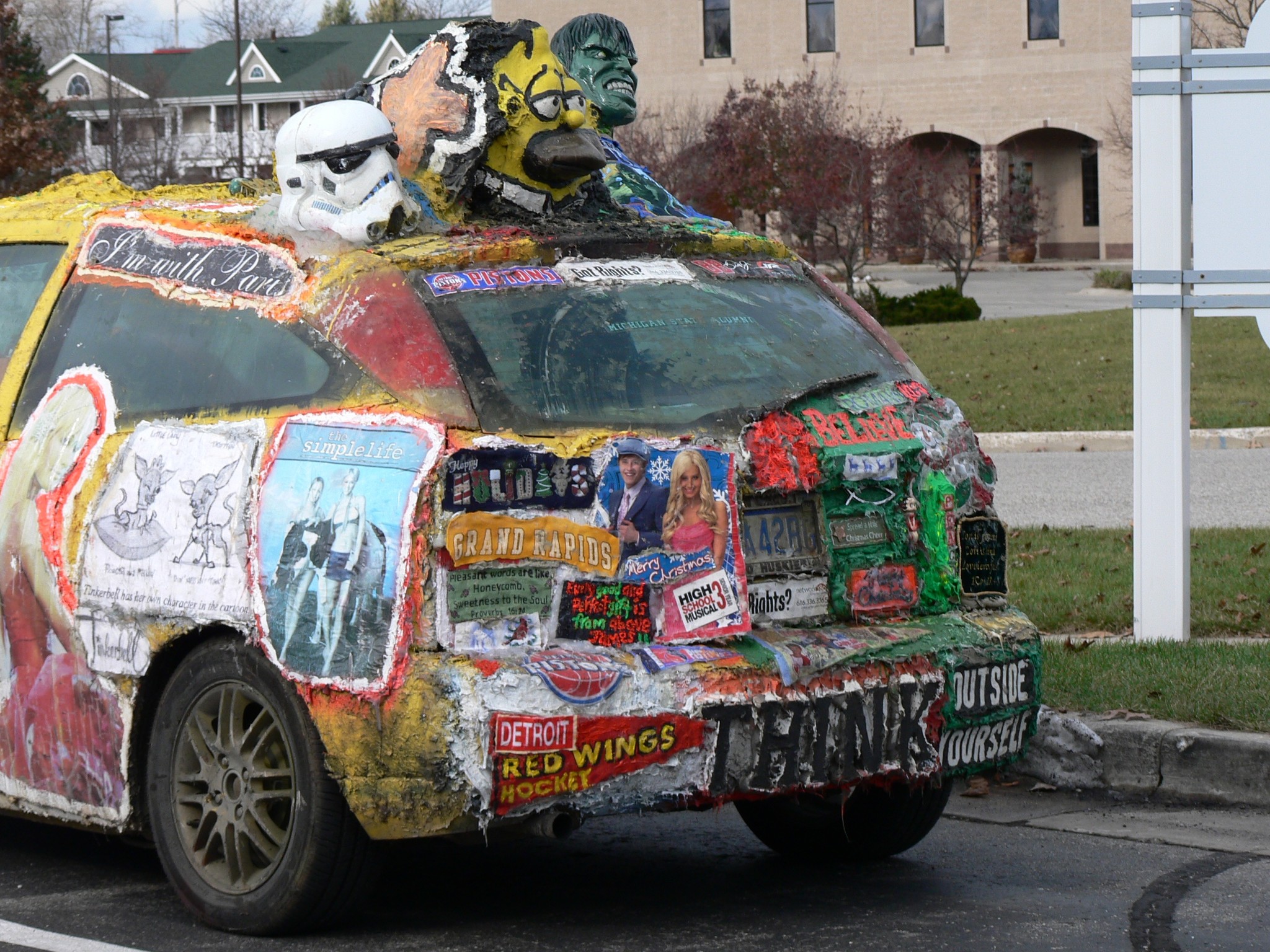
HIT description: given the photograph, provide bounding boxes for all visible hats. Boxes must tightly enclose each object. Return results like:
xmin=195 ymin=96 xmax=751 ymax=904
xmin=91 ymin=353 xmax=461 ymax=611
xmin=617 ymin=438 xmax=651 ymax=461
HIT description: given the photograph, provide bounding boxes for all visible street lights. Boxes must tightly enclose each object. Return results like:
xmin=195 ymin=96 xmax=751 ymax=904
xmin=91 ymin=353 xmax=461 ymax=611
xmin=105 ymin=14 xmax=126 ymax=178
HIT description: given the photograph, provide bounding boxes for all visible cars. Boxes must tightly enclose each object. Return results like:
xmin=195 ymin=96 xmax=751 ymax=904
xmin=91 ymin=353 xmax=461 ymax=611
xmin=0 ymin=120 xmax=1047 ymax=938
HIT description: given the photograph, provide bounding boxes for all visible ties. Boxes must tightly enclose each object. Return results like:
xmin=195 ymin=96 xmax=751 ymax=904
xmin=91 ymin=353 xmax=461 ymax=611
xmin=619 ymin=493 xmax=631 ymax=529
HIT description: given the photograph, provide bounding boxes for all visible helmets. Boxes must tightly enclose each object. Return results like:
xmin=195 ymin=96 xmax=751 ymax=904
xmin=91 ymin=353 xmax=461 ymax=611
xmin=275 ymin=98 xmax=423 ymax=245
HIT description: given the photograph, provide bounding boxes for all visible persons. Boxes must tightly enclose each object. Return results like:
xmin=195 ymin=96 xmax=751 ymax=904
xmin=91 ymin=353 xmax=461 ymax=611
xmin=566 ymin=438 xmax=668 ymax=642
xmin=551 ymin=13 xmax=735 ymax=231
xmin=1 ymin=363 xmax=125 ymax=815
xmin=268 ymin=467 xmax=366 ymax=676
xmin=653 ymin=449 xmax=729 ymax=636
